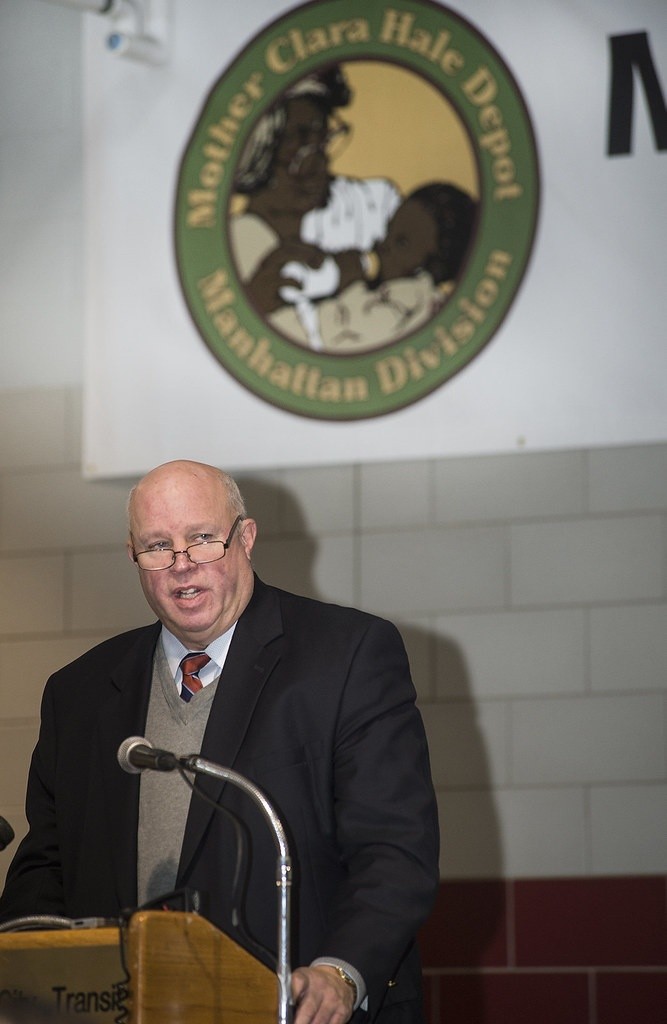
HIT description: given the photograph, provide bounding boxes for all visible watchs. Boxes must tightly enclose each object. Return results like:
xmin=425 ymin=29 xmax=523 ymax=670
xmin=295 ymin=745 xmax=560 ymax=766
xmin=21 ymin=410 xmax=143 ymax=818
xmin=332 ymin=964 xmax=358 ymax=1004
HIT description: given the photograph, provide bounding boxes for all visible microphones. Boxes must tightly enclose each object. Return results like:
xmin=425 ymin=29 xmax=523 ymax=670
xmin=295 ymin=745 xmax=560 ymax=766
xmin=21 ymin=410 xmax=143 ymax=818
xmin=115 ymin=737 xmax=179 ymax=776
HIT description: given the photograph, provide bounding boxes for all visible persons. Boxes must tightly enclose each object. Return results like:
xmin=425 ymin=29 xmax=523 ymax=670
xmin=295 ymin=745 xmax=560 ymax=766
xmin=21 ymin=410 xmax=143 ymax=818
xmin=0 ymin=460 xmax=442 ymax=1024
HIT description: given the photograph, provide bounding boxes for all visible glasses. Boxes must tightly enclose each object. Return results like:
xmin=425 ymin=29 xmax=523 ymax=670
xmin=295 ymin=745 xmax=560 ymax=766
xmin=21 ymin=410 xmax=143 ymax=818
xmin=130 ymin=514 xmax=246 ymax=570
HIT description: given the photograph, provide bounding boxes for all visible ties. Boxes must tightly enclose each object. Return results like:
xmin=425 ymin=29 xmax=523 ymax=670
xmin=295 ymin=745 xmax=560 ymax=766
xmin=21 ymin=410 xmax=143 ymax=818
xmin=178 ymin=651 xmax=211 ymax=703
